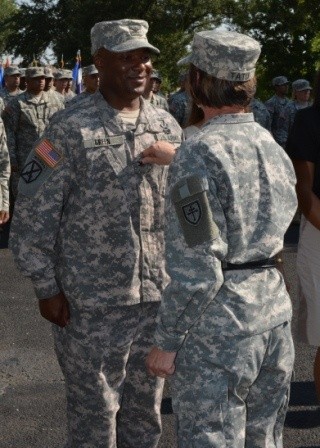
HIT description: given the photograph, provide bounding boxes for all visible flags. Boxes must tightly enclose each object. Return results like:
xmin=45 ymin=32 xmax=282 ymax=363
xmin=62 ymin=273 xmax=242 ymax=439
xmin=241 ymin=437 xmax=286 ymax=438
xmin=72 ymin=58 xmax=82 ymax=94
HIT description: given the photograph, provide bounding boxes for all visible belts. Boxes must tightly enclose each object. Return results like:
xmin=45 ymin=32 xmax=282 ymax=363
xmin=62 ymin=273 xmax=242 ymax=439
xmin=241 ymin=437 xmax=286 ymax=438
xmin=221 ymin=256 xmax=277 ymax=271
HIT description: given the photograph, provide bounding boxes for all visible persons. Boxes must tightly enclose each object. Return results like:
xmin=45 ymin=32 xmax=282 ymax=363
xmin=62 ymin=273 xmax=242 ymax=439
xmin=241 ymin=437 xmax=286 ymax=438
xmin=0 ymin=60 xmax=316 ymax=251
xmin=137 ymin=29 xmax=298 ymax=448
xmin=9 ymin=18 xmax=182 ymax=448
xmin=286 ymin=104 xmax=320 ymax=404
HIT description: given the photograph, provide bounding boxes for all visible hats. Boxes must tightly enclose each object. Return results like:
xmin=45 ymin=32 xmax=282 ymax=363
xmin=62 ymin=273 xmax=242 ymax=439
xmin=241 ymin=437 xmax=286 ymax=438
xmin=54 ymin=69 xmax=69 ymax=80
xmin=150 ymin=68 xmax=160 ymax=80
xmin=89 ymin=19 xmax=161 ymax=56
xmin=176 ymin=29 xmax=261 ymax=82
xmin=292 ymin=79 xmax=312 ymax=92
xmin=65 ymin=70 xmax=72 ymax=79
xmin=25 ymin=67 xmax=46 ymax=78
xmin=44 ymin=67 xmax=54 ymax=79
xmin=19 ymin=68 xmax=26 ymax=78
xmin=83 ymin=64 xmax=98 ymax=76
xmin=272 ymin=76 xmax=290 ymax=86
xmin=4 ymin=67 xmax=21 ymax=76
